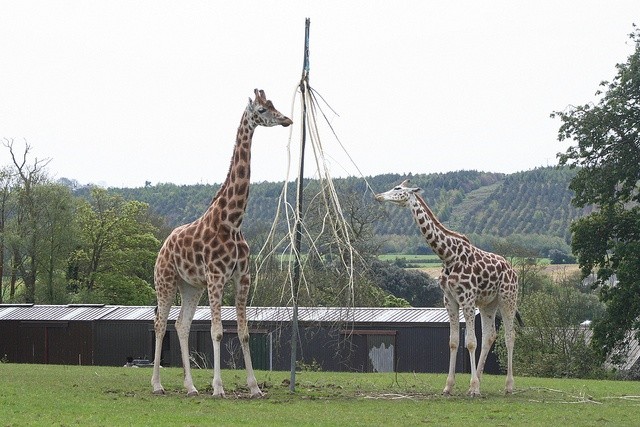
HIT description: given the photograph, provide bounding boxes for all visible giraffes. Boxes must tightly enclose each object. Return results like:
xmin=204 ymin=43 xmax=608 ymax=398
xmin=150 ymin=89 xmax=293 ymax=400
xmin=375 ymin=179 xmax=518 ymax=396
